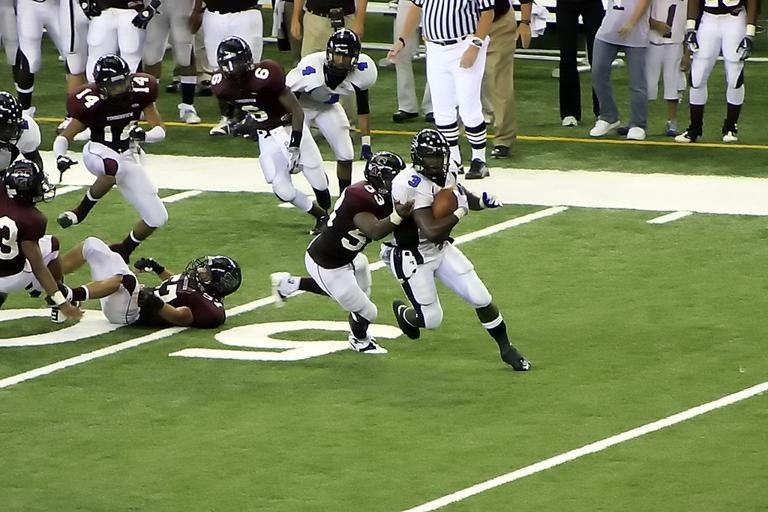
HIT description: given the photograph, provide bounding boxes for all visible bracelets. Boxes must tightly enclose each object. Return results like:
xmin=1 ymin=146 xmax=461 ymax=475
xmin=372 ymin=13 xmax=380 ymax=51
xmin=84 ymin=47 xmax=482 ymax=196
xmin=397 ymin=37 xmax=406 ymax=47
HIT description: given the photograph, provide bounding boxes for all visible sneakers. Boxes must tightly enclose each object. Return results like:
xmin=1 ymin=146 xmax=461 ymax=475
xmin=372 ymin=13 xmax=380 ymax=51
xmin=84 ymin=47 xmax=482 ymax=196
xmin=453 ymin=159 xmax=464 ymax=173
xmin=269 ymin=271 xmax=291 ymax=309
xmin=485 ymin=113 xmax=494 ymax=124
xmin=665 ymin=119 xmax=681 ymax=137
xmin=348 ymin=332 xmax=388 ymax=354
xmin=177 ymin=102 xmax=201 ymax=124
xmin=560 ymin=116 xmax=579 ymax=128
xmin=424 ymin=113 xmax=435 ymax=122
xmin=56 ymin=209 xmax=87 ymax=229
xmin=309 ymin=214 xmax=329 ymax=236
xmin=721 ymin=118 xmax=739 ymax=142
xmin=392 ymin=108 xmax=419 ymax=121
xmin=500 ymin=343 xmax=532 ymax=372
xmin=21 ymin=106 xmax=35 ymax=118
xmin=464 ymin=158 xmax=490 ymax=180
xmin=489 ymin=144 xmax=512 ymax=159
xmin=674 ymin=129 xmax=705 ymax=142
xmin=626 ymin=126 xmax=647 ymax=141
xmin=50 ymin=300 xmax=81 ymax=324
xmin=197 ymin=79 xmax=214 ymax=96
xmin=44 ymin=283 xmax=73 ymax=308
xmin=25 ymin=283 xmax=43 ymax=300
xmin=74 ymin=128 xmax=91 ymax=141
xmin=616 ymin=127 xmax=630 ymax=136
xmin=392 ymin=299 xmax=421 ymax=340
xmin=588 ymin=119 xmax=621 ymax=137
xmin=109 ymin=242 xmax=131 ymax=264
xmin=58 ymin=117 xmax=72 ymax=132
xmin=140 ymin=101 xmax=156 ymax=121
xmin=208 ymin=116 xmax=227 ymax=136
xmin=165 ymin=80 xmax=184 ymax=93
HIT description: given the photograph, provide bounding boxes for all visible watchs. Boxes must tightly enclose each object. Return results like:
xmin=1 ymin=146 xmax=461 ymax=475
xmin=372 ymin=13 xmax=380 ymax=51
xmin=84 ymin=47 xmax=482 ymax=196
xmin=472 ymin=39 xmax=485 ymax=48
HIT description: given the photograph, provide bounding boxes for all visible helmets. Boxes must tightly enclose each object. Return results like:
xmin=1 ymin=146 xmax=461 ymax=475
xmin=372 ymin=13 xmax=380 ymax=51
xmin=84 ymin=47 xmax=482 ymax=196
xmin=325 ymin=29 xmax=361 ymax=74
xmin=2 ymin=159 xmax=56 ymax=208
xmin=183 ymin=254 xmax=242 ymax=299
xmin=216 ymin=35 xmax=254 ymax=81
xmin=363 ymin=150 xmax=406 ymax=198
xmin=410 ymin=127 xmax=451 ymax=188
xmin=92 ymin=53 xmax=131 ymax=99
xmin=0 ymin=90 xmax=29 ymax=152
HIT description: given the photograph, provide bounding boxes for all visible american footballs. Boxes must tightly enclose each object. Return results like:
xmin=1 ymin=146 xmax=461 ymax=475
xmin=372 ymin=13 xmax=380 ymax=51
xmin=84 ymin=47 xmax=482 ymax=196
xmin=431 ymin=189 xmax=458 ymax=220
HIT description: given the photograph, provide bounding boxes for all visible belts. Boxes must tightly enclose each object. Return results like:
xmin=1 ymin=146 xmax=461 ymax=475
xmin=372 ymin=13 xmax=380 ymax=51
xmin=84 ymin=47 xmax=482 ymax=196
xmin=424 ymin=38 xmax=468 ymax=48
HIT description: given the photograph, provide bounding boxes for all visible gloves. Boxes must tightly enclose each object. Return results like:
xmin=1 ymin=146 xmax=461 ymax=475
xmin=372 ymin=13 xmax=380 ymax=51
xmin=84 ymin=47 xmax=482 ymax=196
xmin=131 ymin=4 xmax=161 ymax=31
xmin=479 ymin=191 xmax=503 ymax=209
xmin=138 ymin=290 xmax=164 ymax=314
xmin=736 ymin=35 xmax=754 ymax=61
xmin=133 ymin=257 xmax=166 ymax=275
xmin=286 ymin=146 xmax=303 ymax=174
xmin=78 ymin=0 xmax=101 ymax=20
xmin=128 ymin=127 xmax=145 ymax=143
xmin=454 ymin=183 xmax=470 ymax=214
xmin=682 ymin=28 xmax=699 ymax=53
xmin=55 ymin=155 xmax=79 ymax=182
xmin=359 ymin=144 xmax=373 ymax=161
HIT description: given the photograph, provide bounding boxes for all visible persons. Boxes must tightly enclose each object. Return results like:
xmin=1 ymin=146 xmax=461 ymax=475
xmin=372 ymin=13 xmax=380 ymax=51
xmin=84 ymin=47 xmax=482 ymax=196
xmin=142 ymin=1 xmax=206 ymax=127
xmin=390 ymin=128 xmax=532 ymax=372
xmin=289 ymin=1 xmax=368 ymax=132
xmin=285 ymin=28 xmax=377 ymax=198
xmin=556 ymin=0 xmax=608 ymax=128
xmin=269 ymin=151 xmax=411 ymax=356
xmin=190 ymin=1 xmax=262 ymax=136
xmin=54 ymin=52 xmax=167 ymax=264
xmin=482 ymin=0 xmax=531 ymax=161
xmin=11 ymin=0 xmax=74 ymax=122
xmin=25 ymin=238 xmax=242 ymax=328
xmin=210 ymin=34 xmax=332 ymax=237
xmin=0 ymin=1 xmax=18 ymax=92
xmin=643 ymin=1 xmax=693 ymax=138
xmin=387 ymin=1 xmax=497 ymax=179
xmin=672 ymin=0 xmax=756 ymax=143
xmin=390 ymin=0 xmax=447 ymax=123
xmin=80 ymin=1 xmax=159 ymax=85
xmin=57 ymin=0 xmax=94 ymax=137
xmin=0 ymin=161 xmax=84 ymax=323
xmin=0 ymin=86 xmax=49 ymax=204
xmin=588 ymin=1 xmax=649 ymax=141
